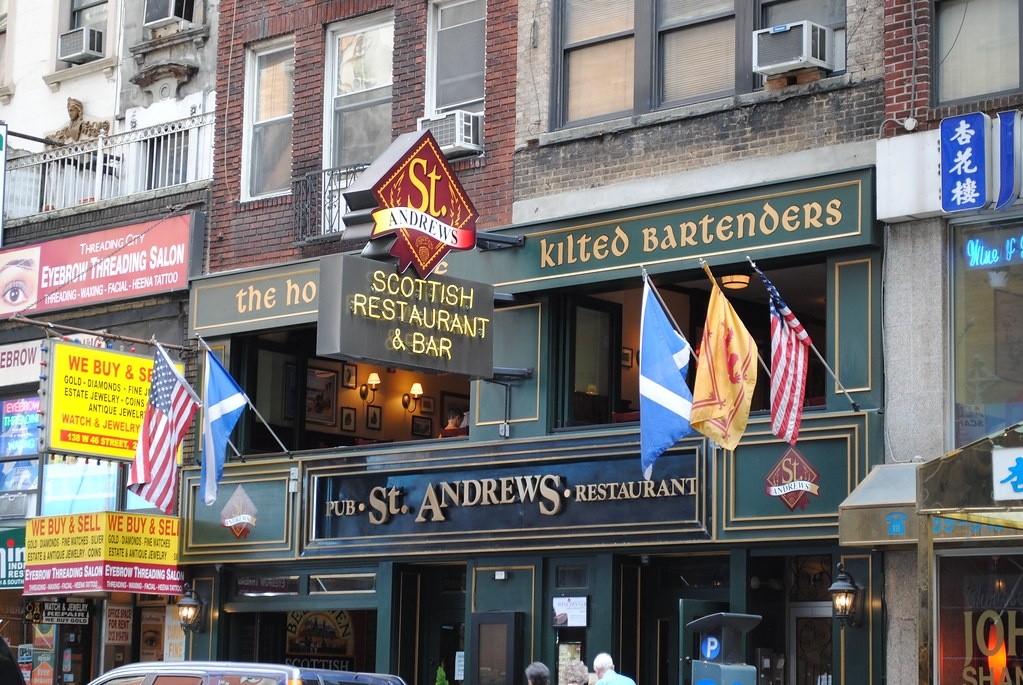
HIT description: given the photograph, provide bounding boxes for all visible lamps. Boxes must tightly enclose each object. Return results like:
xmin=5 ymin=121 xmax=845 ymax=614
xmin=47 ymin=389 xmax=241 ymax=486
xmin=722 ymin=274 xmax=751 ymax=289
xmin=360 ymin=373 xmax=380 ymax=404
xmin=403 ymin=382 xmax=423 ymax=413
xmin=828 ymin=561 xmax=866 ymax=629
xmin=177 ymin=582 xmax=207 ymax=633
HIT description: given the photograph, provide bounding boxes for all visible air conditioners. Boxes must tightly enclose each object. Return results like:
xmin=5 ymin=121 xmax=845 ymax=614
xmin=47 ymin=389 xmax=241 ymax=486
xmin=143 ymin=0 xmax=190 ymax=30
xmin=415 ymin=109 xmax=483 ymax=159
xmin=58 ymin=25 xmax=105 ymax=64
xmin=751 ymin=19 xmax=835 ymax=74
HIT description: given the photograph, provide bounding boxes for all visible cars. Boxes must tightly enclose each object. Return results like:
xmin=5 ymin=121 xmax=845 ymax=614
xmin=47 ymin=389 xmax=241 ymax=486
xmin=86 ymin=661 xmax=406 ymax=685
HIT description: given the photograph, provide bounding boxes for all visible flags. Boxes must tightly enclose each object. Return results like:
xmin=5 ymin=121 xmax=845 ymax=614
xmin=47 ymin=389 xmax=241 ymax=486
xmin=638 ymin=278 xmax=693 ymax=479
xmin=200 ymin=346 xmax=249 ymax=508
xmin=751 ymin=264 xmax=811 ymax=446
xmin=690 ymin=262 xmax=758 ymax=451
xmin=125 ymin=342 xmax=201 ymax=515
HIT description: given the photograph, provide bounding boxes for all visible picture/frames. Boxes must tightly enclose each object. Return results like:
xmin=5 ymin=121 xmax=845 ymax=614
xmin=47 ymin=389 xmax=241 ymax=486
xmin=367 ymin=405 xmax=382 ymax=431
xmin=282 ymin=361 xmax=338 ymax=427
xmin=341 ymin=407 xmax=356 ymax=432
xmin=342 ymin=363 xmax=357 ymax=390
xmin=992 ymin=289 xmax=1023 ymax=383
xmin=622 ymin=347 xmax=633 ymax=368
xmin=439 ymin=390 xmax=470 ymax=432
xmin=420 ymin=395 xmax=435 ymax=416
xmin=412 ymin=415 xmax=432 ymax=438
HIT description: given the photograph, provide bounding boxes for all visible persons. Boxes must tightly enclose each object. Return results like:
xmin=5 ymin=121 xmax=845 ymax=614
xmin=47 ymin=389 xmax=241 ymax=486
xmin=562 ymin=661 xmax=588 ymax=685
xmin=525 ymin=662 xmax=550 ymax=685
xmin=0 ymin=247 xmax=39 ymax=314
xmin=67 ymin=97 xmax=83 ymax=141
xmin=141 ymin=624 xmax=161 ymax=650
xmin=593 ymin=653 xmax=635 ymax=685
